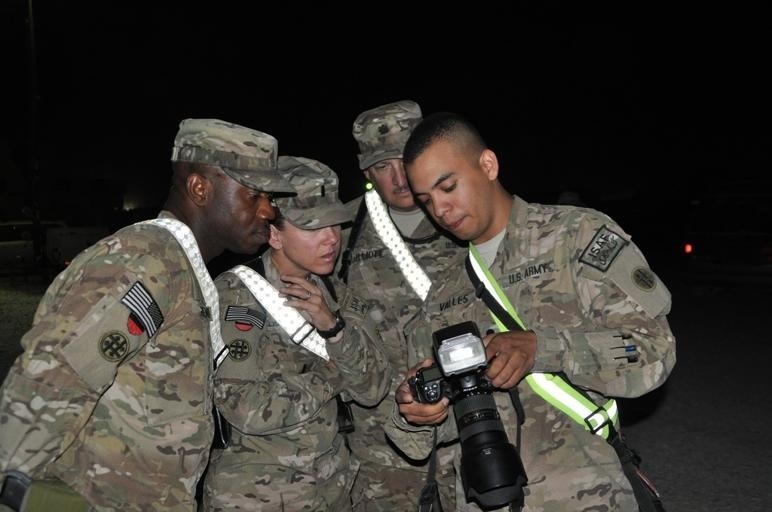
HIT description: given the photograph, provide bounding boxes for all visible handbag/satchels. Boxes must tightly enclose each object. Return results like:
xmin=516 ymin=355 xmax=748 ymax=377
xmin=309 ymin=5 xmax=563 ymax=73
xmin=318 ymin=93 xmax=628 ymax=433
xmin=615 ymin=430 xmax=665 ymax=512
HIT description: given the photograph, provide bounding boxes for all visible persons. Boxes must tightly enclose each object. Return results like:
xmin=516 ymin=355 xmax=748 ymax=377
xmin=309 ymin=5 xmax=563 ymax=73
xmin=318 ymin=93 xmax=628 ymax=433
xmin=199 ymin=155 xmax=391 ymax=512
xmin=378 ymin=110 xmax=676 ymax=510
xmin=330 ymin=97 xmax=465 ymax=512
xmin=0 ymin=117 xmax=296 ymax=511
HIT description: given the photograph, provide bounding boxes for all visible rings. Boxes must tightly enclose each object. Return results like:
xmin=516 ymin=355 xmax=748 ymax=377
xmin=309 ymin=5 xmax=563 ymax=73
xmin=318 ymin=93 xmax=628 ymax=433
xmin=304 ymin=291 xmax=312 ymax=300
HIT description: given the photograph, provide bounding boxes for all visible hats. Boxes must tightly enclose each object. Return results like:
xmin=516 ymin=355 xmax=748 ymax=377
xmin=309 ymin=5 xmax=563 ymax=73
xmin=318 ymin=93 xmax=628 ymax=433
xmin=170 ymin=117 xmax=297 ymax=198
xmin=277 ymin=155 xmax=355 ymax=231
xmin=353 ymin=100 xmax=423 ymax=171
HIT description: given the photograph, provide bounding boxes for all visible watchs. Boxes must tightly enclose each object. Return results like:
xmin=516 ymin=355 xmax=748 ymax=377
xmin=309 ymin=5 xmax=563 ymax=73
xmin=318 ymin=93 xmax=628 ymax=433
xmin=313 ymin=307 xmax=347 ymax=340
